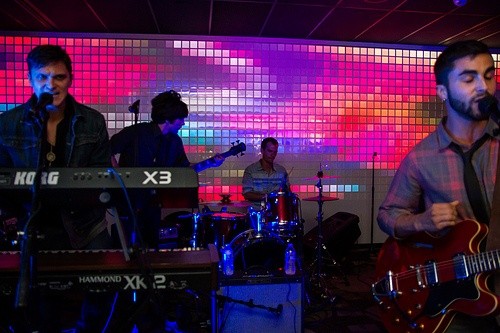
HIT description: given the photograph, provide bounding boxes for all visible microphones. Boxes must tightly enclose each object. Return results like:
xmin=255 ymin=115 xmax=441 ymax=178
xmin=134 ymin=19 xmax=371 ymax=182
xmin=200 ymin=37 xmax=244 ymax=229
xmin=25 ymin=91 xmax=52 ymax=119
xmin=277 ymin=303 xmax=283 ymax=314
xmin=477 ymin=96 xmax=500 ymax=127
xmin=316 ymin=163 xmax=324 ymax=179
xmin=128 ymin=100 xmax=139 ymax=113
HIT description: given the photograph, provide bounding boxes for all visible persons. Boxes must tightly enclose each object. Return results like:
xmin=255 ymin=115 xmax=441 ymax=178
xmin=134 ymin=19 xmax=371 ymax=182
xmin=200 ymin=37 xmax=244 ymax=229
xmin=241 ymin=137 xmax=294 ymax=217
xmin=0 ymin=45 xmax=108 ymax=333
xmin=376 ymin=39 xmax=500 ymax=251
xmin=109 ymin=91 xmax=225 ymax=248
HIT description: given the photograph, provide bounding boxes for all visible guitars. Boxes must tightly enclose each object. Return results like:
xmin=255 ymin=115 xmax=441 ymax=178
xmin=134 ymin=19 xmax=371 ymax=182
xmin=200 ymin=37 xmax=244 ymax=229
xmin=373 ymin=219 xmax=500 ymax=333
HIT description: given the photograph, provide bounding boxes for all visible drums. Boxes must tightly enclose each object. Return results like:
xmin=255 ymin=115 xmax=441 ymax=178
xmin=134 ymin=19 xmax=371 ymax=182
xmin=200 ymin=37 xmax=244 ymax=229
xmin=263 ymin=192 xmax=299 ymax=233
xmin=202 ymin=211 xmax=247 ymax=247
xmin=225 ymin=230 xmax=303 ymax=277
xmin=235 ymin=204 xmax=271 ymax=240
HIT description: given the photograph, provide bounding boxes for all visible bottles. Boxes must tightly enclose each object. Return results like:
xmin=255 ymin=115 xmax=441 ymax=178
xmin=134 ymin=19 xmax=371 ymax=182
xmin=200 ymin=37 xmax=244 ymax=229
xmin=284 ymin=243 xmax=297 ymax=275
xmin=224 ymin=245 xmax=234 ymax=275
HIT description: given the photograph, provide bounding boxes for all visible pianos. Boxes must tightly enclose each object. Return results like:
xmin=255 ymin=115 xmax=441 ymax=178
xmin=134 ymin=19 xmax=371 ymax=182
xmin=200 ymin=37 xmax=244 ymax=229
xmin=0 ymin=244 xmax=221 ymax=333
xmin=0 ymin=167 xmax=200 ymax=209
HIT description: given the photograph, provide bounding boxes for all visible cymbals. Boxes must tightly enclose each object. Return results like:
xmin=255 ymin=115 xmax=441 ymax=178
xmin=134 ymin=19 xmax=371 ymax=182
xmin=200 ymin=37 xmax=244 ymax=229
xmin=198 ymin=200 xmax=251 ymax=208
xmin=303 ymin=194 xmax=338 ymax=204
xmin=302 ymin=173 xmax=342 ymax=182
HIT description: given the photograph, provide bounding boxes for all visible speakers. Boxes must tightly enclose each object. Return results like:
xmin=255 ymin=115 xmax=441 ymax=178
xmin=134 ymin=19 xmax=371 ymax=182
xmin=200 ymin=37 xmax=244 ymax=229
xmin=305 ymin=210 xmax=361 ymax=247
xmin=211 ymin=282 xmax=302 ymax=333
